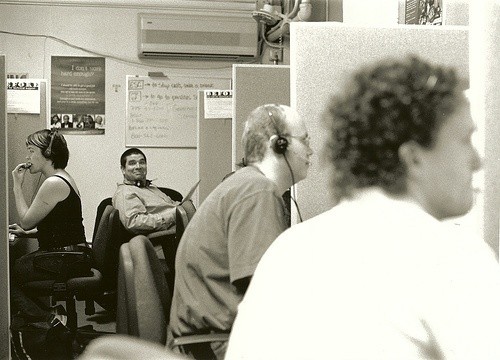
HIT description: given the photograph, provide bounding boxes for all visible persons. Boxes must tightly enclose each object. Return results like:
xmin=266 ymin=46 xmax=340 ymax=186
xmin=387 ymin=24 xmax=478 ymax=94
xmin=84 ymin=115 xmax=95 ymax=128
xmin=51 ymin=115 xmax=61 ymax=128
xmin=9 ymin=129 xmax=92 ymax=353
xmin=95 ymin=116 xmax=105 ymax=129
xmin=166 ymin=105 xmax=314 ymax=360
xmin=73 ymin=116 xmax=84 ymax=128
xmin=223 ymin=54 xmax=500 ymax=360
xmin=61 ymin=115 xmax=73 ymax=128
xmin=112 ymin=147 xmax=196 ymax=232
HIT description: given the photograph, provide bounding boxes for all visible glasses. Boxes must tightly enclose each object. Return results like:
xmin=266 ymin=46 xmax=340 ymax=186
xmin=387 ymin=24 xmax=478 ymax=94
xmin=279 ymin=134 xmax=310 ymax=148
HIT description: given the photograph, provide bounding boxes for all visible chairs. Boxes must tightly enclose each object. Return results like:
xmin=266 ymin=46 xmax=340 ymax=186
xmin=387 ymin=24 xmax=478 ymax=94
xmin=8 ymin=187 xmax=230 ymax=360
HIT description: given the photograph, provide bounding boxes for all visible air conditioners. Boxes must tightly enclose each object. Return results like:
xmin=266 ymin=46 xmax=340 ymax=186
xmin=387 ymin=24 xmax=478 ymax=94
xmin=137 ymin=14 xmax=260 ymax=61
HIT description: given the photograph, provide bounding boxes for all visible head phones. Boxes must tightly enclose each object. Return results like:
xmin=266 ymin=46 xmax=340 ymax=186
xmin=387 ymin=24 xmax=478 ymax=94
xmin=125 ymin=180 xmax=150 ymax=187
xmin=264 ymin=105 xmax=288 ymax=154
xmin=43 ymin=131 xmax=56 ymax=158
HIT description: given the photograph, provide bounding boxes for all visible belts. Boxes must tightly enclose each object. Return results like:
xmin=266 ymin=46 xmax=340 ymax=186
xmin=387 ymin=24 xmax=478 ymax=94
xmin=52 ymin=245 xmax=91 ymax=253
